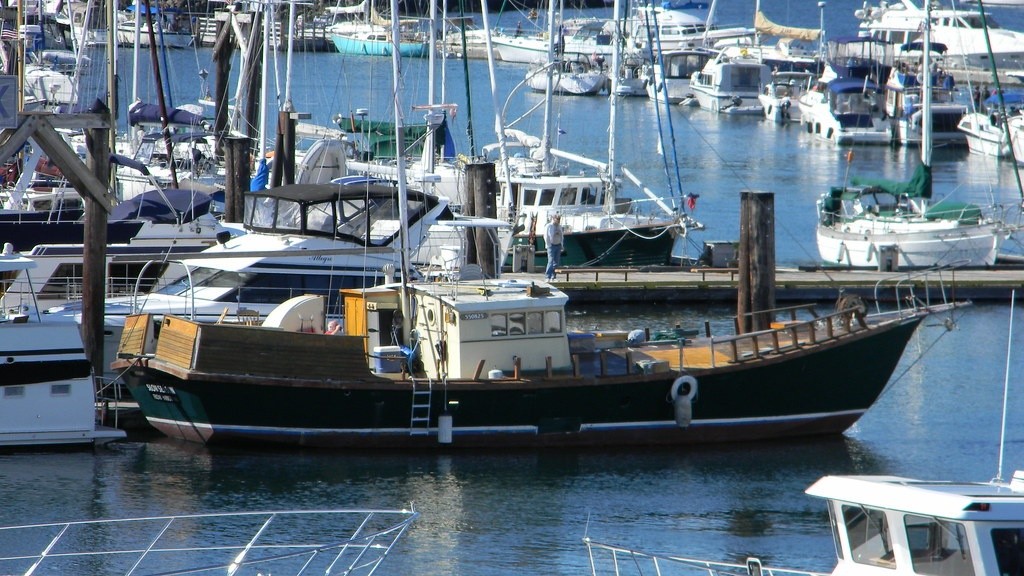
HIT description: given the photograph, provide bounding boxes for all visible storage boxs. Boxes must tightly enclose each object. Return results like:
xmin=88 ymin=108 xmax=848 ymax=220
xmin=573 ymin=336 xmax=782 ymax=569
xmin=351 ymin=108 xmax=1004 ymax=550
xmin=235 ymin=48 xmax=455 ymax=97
xmin=374 ymin=345 xmax=401 ymax=373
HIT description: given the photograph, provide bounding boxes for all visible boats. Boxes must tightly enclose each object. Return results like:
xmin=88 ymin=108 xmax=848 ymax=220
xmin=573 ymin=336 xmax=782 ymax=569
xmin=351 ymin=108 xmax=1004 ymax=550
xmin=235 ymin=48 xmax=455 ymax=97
xmin=1 ymin=0 xmax=1022 ymax=575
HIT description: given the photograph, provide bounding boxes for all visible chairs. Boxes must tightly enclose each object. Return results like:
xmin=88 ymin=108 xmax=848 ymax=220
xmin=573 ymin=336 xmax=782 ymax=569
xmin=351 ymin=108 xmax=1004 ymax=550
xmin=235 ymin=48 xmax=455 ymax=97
xmin=216 ymin=307 xmax=229 ymax=325
xmin=236 ymin=310 xmax=259 ymax=326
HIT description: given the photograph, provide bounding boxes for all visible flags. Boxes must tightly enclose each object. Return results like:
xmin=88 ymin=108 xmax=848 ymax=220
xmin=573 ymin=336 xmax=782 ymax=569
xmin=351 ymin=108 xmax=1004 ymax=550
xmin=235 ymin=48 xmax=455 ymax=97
xmin=687 ymin=192 xmax=699 ymax=211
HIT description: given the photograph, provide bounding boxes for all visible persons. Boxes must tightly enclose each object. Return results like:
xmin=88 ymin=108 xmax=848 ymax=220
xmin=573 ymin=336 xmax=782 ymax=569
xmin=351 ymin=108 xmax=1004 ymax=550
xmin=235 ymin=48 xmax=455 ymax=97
xmin=325 ymin=321 xmax=342 ymax=336
xmin=895 ymin=54 xmax=938 ymax=86
xmin=544 ymin=215 xmax=563 ymax=283
xmin=973 ymin=85 xmax=1022 ymax=128
